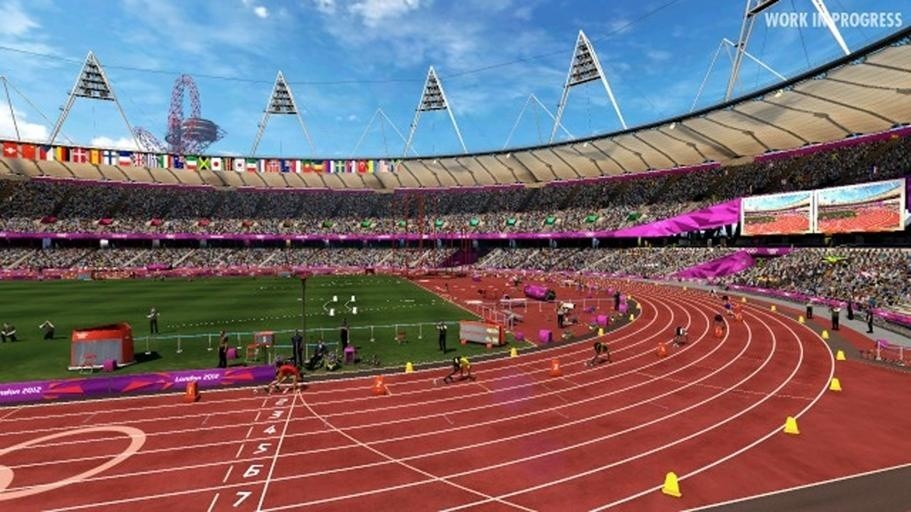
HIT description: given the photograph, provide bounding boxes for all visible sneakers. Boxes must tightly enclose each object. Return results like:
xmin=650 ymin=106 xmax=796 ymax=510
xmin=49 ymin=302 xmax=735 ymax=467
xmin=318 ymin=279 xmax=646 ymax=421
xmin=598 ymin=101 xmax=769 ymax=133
xmin=263 ymin=384 xmax=272 ymax=396
xmin=273 ymin=384 xmax=281 ymax=392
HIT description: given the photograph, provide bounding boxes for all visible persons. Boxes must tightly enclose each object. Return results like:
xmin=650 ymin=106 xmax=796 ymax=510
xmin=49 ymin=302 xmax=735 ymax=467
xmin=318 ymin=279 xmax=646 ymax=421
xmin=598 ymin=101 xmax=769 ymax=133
xmin=290 ymin=328 xmax=305 ymax=367
xmin=340 ymin=321 xmax=350 ymax=352
xmin=0 ymin=128 xmax=911 ymax=237
xmin=216 ymin=329 xmax=229 ymax=368
xmin=584 ymin=342 xmax=612 ymax=365
xmin=443 ymin=356 xmax=476 ymax=384
xmin=673 ymin=326 xmax=692 ymax=349
xmin=305 ymin=337 xmax=326 ymax=369
xmin=1 ymin=245 xmax=911 ymax=343
xmin=433 ymin=318 xmax=450 ymax=353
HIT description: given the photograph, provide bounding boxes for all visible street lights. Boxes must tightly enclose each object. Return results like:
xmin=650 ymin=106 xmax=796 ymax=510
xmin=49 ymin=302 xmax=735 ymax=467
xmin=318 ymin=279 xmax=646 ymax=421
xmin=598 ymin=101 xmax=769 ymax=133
xmin=299 ymin=274 xmax=309 ymax=342
